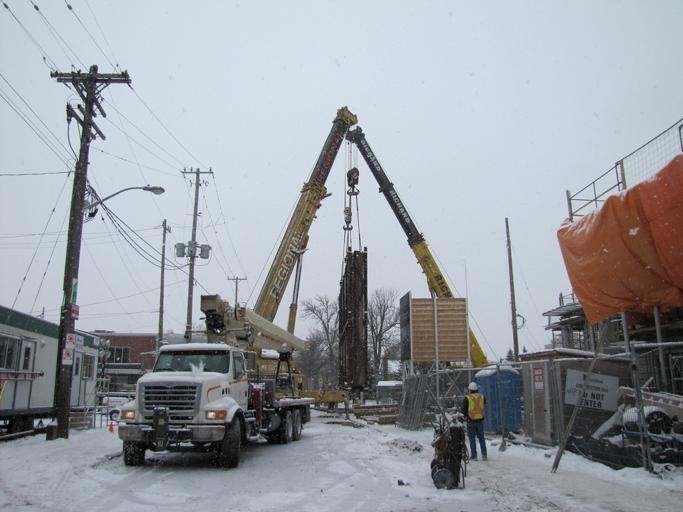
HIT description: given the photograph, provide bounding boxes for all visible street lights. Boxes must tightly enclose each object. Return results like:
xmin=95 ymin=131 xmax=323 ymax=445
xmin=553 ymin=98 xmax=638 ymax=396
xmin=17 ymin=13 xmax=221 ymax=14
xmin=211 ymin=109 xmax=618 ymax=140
xmin=53 ymin=186 xmax=165 ymax=438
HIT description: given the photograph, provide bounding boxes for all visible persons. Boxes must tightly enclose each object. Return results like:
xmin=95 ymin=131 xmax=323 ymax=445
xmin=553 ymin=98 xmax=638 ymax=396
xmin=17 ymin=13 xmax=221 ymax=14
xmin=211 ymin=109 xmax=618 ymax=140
xmin=462 ymin=381 xmax=489 ymax=461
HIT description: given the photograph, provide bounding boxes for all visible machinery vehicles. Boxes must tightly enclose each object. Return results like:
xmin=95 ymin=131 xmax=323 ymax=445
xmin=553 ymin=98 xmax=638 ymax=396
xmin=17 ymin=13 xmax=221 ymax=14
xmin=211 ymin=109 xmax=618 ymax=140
xmin=118 ymin=106 xmax=488 ymax=467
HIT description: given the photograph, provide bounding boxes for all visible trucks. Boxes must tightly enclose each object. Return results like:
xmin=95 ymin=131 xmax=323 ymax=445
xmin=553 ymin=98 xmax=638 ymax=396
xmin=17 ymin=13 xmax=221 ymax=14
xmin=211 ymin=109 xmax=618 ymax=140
xmin=103 ymin=397 xmax=129 ymax=420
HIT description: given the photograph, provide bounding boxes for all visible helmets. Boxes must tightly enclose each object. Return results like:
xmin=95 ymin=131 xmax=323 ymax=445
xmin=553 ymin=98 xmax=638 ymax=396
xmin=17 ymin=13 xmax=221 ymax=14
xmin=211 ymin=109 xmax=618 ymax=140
xmin=468 ymin=382 xmax=478 ymax=391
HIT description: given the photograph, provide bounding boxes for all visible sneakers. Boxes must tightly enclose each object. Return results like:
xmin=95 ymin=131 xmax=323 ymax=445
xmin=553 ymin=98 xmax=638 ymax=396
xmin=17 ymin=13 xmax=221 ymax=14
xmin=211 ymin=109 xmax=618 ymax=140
xmin=481 ymin=454 xmax=488 ymax=462
xmin=470 ymin=455 xmax=478 ymax=460
